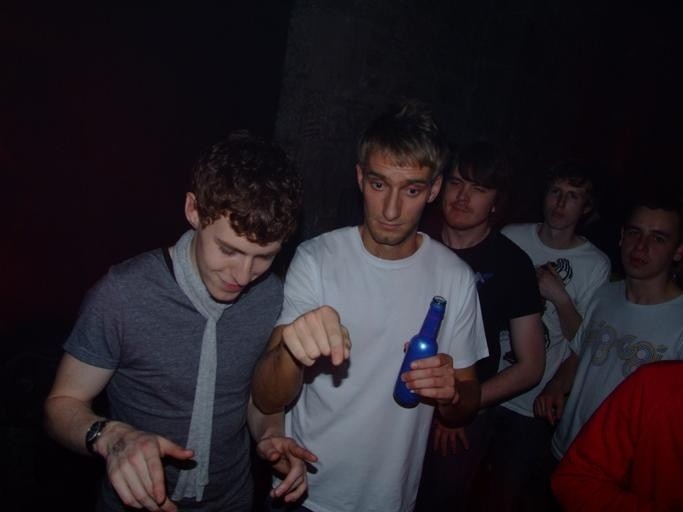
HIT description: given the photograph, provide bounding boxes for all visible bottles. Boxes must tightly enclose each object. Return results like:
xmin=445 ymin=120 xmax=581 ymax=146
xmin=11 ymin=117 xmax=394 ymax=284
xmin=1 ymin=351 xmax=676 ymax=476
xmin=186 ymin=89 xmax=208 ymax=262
xmin=392 ymin=294 xmax=448 ymax=410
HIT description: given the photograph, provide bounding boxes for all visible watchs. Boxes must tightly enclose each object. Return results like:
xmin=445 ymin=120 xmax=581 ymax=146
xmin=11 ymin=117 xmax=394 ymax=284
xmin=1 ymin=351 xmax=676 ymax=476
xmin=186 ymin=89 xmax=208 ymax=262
xmin=85 ymin=417 xmax=112 ymax=453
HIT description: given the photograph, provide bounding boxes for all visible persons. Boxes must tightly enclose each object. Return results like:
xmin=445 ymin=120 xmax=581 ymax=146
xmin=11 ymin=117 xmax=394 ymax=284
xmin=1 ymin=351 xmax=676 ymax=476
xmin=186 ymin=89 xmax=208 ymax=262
xmin=251 ymin=99 xmax=490 ymax=512
xmin=43 ymin=131 xmax=318 ymax=512
